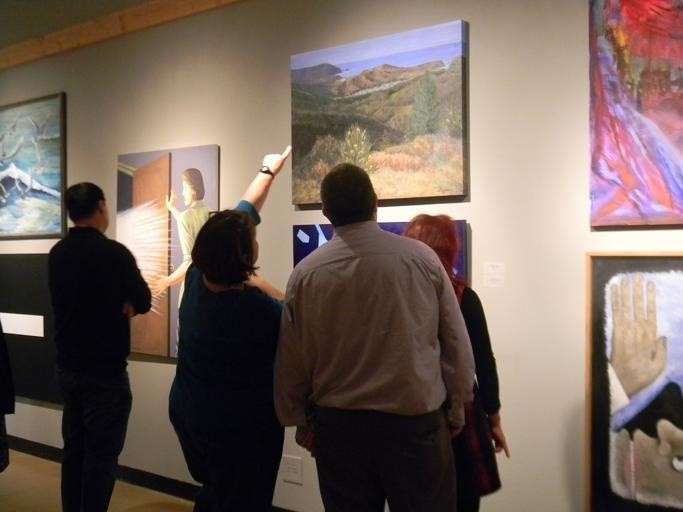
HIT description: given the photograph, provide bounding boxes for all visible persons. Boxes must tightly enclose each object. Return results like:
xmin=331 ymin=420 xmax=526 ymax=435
xmin=42 ymin=181 xmax=152 ymax=512
xmin=166 ymin=145 xmax=292 ymax=512
xmin=154 ymin=168 xmax=216 ymax=359
xmin=400 ymin=213 xmax=510 ymax=511
xmin=272 ymin=163 xmax=476 ymax=512
xmin=606 ymin=274 xmax=682 ymax=509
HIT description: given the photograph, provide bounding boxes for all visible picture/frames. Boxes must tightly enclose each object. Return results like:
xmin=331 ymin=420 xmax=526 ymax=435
xmin=585 ymin=251 xmax=682 ymax=512
xmin=0 ymin=90 xmax=66 ymax=241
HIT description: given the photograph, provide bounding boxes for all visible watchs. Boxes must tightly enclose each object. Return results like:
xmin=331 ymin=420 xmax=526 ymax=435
xmin=259 ymin=166 xmax=274 ymax=180
